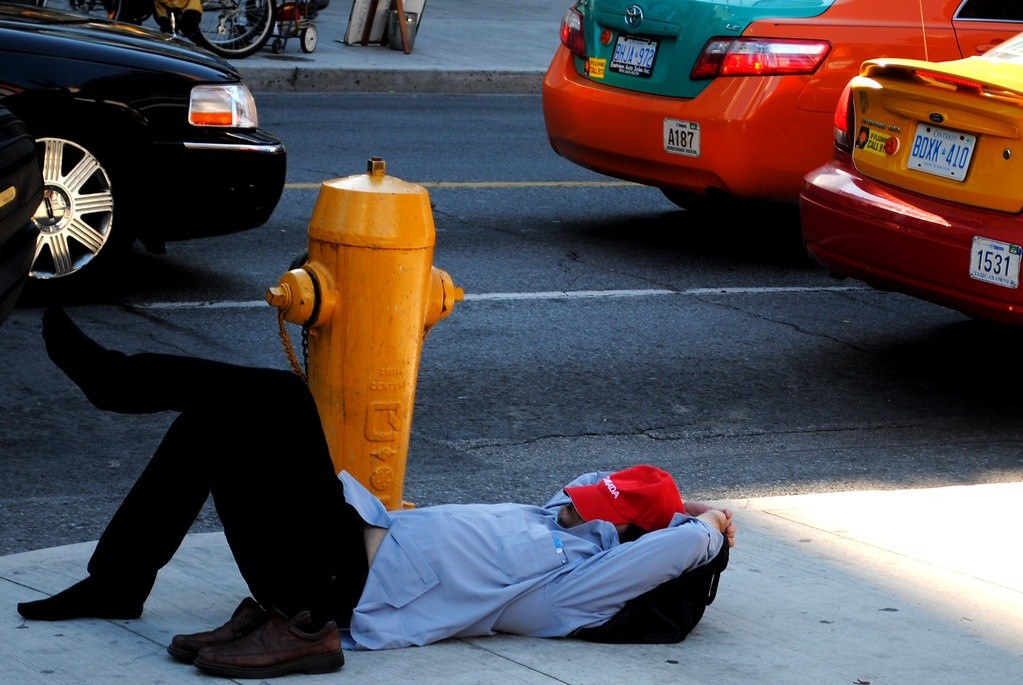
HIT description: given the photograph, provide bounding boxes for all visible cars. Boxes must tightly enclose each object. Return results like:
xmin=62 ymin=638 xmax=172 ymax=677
xmin=0 ymin=0 xmax=289 ymax=305
xmin=541 ymin=0 xmax=1022 ymax=236
xmin=796 ymin=31 xmax=1023 ymax=326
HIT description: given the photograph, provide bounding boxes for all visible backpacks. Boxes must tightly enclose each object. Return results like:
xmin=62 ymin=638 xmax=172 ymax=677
xmin=570 ymin=532 xmax=733 ymax=643
xmin=0 ymin=103 xmax=44 ymax=248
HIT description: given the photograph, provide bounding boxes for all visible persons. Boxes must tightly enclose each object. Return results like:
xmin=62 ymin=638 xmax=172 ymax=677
xmin=15 ymin=299 xmax=737 ymax=655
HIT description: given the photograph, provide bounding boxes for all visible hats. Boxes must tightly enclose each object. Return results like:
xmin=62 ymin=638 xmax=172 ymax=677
xmin=561 ymin=465 xmax=687 ymax=533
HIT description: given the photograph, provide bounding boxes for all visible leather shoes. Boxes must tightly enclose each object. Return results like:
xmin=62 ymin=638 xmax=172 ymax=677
xmin=167 ymin=594 xmax=345 ymax=678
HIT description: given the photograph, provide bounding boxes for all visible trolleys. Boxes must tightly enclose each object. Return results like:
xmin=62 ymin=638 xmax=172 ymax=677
xmin=242 ymin=0 xmax=317 ymax=55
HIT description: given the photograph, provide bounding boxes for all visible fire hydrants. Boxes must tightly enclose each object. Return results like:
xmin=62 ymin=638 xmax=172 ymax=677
xmin=268 ymin=154 xmax=467 ymax=517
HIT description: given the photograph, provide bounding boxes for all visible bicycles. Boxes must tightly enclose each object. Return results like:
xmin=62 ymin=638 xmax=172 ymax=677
xmin=35 ymin=1 xmax=276 ymax=60
xmin=176 ymin=1 xmax=266 ymax=47
